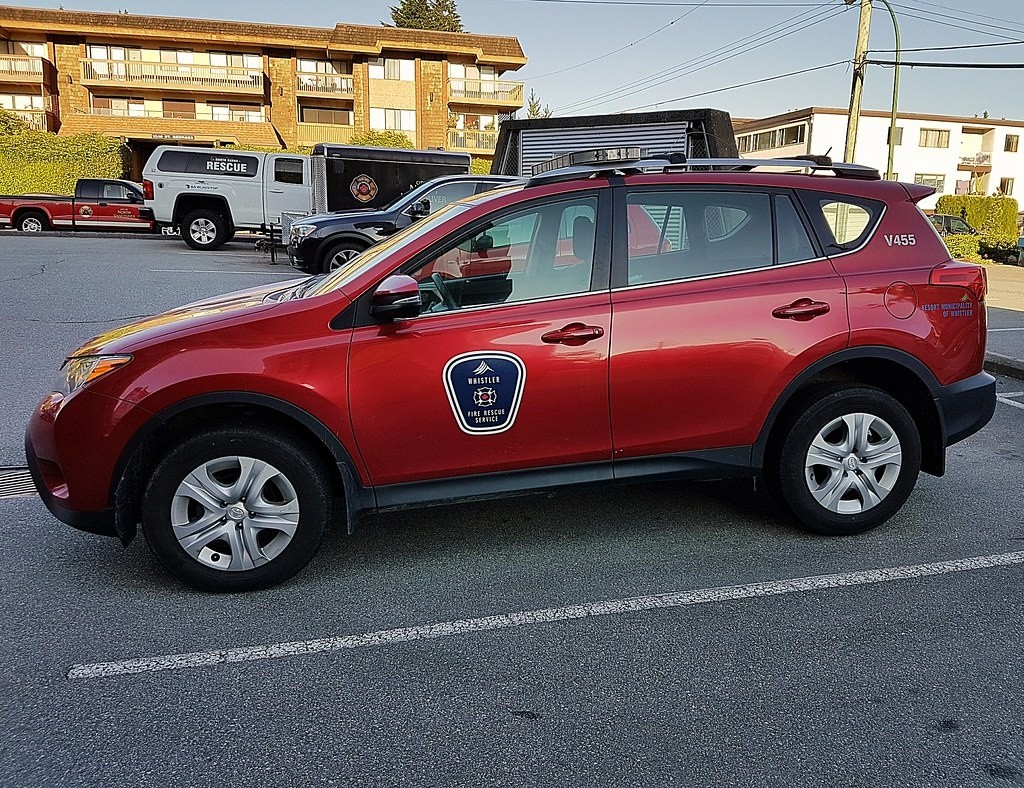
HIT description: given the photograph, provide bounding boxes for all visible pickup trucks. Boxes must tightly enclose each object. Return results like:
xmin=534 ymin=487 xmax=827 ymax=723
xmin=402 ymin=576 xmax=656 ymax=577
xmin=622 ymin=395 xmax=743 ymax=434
xmin=0 ymin=176 xmax=160 ymax=233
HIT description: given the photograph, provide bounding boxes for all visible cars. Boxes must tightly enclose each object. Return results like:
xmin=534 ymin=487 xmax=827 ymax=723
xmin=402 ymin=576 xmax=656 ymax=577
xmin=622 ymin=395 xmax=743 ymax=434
xmin=409 ymin=201 xmax=674 ymax=286
xmin=927 ymin=212 xmax=979 ymax=238
xmin=135 ymin=144 xmax=312 ymax=250
xmin=284 ymin=175 xmax=532 ymax=278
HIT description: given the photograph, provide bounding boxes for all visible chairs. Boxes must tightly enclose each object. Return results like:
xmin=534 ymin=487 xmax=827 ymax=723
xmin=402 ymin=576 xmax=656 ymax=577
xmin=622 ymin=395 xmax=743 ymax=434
xmin=563 ymin=216 xmax=596 ymax=292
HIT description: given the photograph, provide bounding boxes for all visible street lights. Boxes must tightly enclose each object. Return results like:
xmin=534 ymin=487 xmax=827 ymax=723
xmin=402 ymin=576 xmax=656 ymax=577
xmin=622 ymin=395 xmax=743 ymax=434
xmin=846 ymin=0 xmax=898 ymax=182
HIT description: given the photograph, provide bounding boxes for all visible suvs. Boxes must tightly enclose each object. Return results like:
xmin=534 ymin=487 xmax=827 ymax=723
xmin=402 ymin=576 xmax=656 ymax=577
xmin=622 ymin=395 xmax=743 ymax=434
xmin=23 ymin=145 xmax=998 ymax=592
